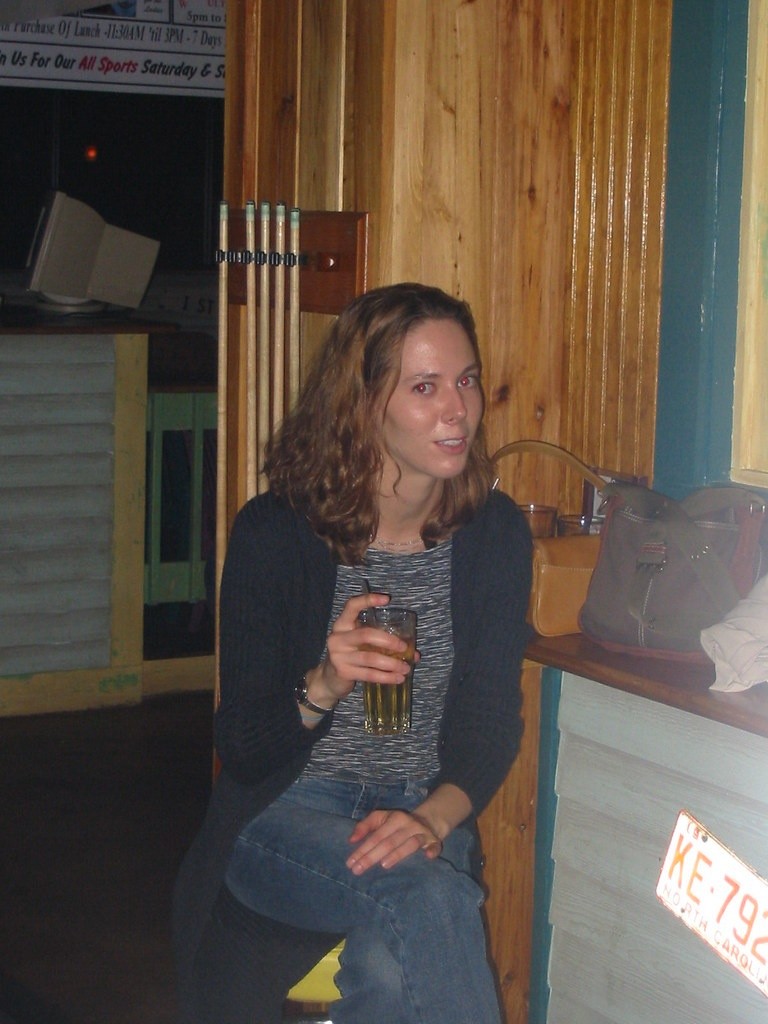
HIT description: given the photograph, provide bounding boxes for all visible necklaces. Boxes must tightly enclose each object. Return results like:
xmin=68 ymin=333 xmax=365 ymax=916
xmin=369 ymin=532 xmax=424 ymax=552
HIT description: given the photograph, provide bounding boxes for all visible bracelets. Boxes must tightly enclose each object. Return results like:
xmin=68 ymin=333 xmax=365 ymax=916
xmin=300 ymin=713 xmax=325 ymax=722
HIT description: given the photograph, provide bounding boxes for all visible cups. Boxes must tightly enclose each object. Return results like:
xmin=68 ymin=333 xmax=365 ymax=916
xmin=355 ymin=606 xmax=417 ymax=735
xmin=556 ymin=514 xmax=604 ymax=538
xmin=517 ymin=505 xmax=557 ymax=539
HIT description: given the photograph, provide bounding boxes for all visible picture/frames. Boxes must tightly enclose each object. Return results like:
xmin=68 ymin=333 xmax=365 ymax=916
xmin=581 ymin=466 xmax=638 ymax=535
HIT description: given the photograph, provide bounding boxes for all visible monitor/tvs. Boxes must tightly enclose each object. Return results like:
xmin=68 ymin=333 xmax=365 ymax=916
xmin=20 ymin=191 xmax=161 ymax=314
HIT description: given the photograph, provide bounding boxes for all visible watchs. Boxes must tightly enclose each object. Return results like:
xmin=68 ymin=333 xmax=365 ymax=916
xmin=295 ymin=672 xmax=335 ymax=716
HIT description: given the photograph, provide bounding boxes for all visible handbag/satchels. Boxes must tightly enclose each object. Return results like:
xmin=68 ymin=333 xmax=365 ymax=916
xmin=577 ymin=481 xmax=768 ymax=665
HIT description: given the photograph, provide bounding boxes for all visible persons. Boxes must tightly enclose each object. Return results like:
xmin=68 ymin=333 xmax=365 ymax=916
xmin=169 ymin=282 xmax=533 ymax=1024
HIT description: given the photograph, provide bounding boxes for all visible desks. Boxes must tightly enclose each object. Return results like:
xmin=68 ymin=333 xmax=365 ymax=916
xmin=521 ymin=621 xmax=768 ymax=740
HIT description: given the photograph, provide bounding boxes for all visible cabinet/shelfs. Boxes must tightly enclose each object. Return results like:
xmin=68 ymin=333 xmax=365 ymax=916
xmin=0 ymin=299 xmax=180 ymax=721
